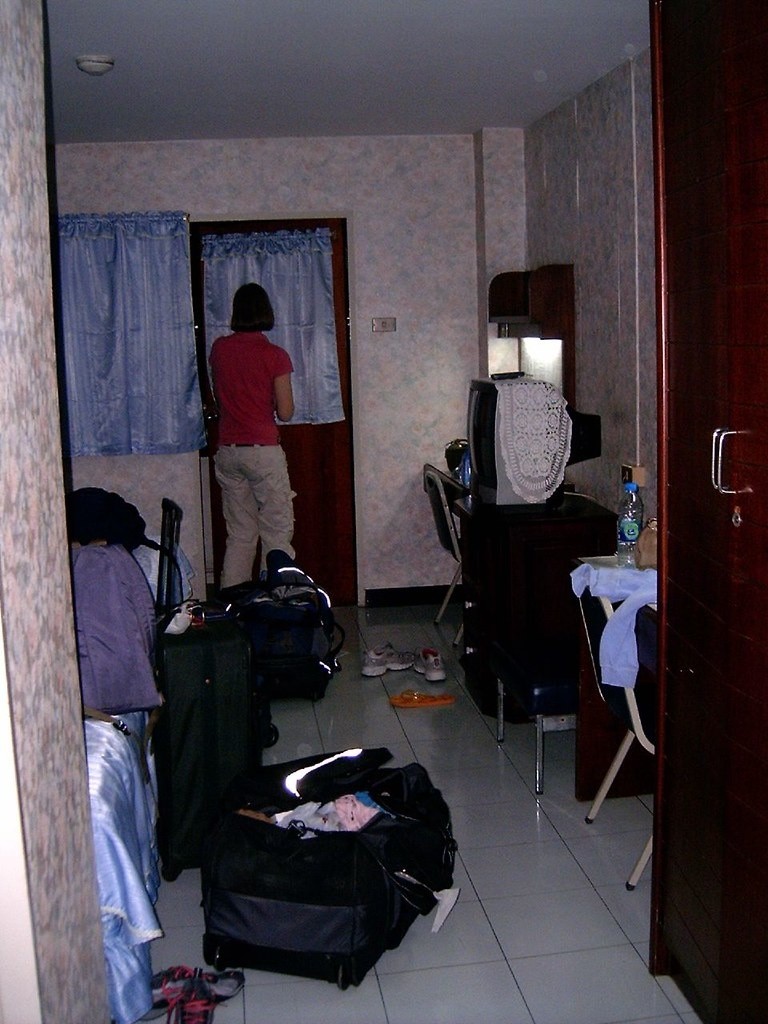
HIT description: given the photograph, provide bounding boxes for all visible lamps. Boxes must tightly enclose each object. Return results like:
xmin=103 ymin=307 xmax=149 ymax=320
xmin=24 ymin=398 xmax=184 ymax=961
xmin=75 ymin=55 xmax=115 ymax=76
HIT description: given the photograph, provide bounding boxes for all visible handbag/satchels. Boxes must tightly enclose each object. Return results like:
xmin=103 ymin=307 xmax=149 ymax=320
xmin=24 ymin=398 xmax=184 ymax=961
xmin=68 ymin=539 xmax=165 ymax=783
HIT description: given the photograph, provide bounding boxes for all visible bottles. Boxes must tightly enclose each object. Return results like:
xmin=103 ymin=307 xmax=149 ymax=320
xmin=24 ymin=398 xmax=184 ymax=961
xmin=463 ymin=449 xmax=471 ymax=489
xmin=617 ymin=483 xmax=643 ymax=570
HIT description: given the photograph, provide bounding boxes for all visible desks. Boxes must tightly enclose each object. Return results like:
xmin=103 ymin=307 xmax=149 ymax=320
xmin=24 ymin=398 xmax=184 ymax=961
xmin=571 ymin=558 xmax=659 ymax=802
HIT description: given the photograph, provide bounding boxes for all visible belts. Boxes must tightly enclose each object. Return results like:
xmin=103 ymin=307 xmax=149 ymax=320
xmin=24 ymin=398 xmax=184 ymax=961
xmin=224 ymin=444 xmax=266 ymax=447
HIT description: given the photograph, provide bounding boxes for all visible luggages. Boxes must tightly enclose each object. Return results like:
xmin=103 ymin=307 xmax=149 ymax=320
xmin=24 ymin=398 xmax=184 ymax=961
xmin=199 ymin=747 xmax=459 ymax=989
xmin=157 ymin=496 xmax=271 ymax=881
xmin=212 ymin=549 xmax=345 ymax=702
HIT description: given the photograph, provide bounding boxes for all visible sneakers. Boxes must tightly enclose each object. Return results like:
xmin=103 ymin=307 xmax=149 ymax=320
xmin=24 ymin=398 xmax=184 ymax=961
xmin=362 ymin=642 xmax=416 ymax=678
xmin=412 ymin=647 xmax=447 ymax=682
xmin=141 ymin=966 xmax=248 ymax=1024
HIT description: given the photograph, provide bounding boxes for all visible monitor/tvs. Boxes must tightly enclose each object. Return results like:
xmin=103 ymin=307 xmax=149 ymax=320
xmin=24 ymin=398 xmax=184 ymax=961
xmin=467 ymin=377 xmax=602 ymax=504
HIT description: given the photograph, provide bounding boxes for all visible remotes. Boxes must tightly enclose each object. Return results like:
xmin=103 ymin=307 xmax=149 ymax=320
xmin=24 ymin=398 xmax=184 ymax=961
xmin=491 ymin=372 xmax=525 ymax=380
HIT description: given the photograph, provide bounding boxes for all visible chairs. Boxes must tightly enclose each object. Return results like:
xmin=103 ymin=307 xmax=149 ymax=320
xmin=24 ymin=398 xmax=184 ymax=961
xmin=425 ymin=471 xmax=465 ymax=647
xmin=578 ymin=557 xmax=659 ymax=892
xmin=156 ymin=497 xmax=184 ymax=612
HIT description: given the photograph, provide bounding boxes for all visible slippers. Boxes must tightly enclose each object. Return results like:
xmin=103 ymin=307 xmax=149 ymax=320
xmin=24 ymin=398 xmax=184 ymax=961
xmin=391 ymin=689 xmax=456 ymax=709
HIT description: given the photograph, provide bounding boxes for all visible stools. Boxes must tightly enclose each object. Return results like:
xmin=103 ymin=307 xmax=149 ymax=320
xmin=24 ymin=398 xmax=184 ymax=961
xmin=487 ymin=632 xmax=578 ymax=795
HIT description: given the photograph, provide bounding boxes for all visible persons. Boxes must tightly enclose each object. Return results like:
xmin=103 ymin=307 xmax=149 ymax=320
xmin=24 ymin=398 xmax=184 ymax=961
xmin=208 ymin=283 xmax=297 ymax=592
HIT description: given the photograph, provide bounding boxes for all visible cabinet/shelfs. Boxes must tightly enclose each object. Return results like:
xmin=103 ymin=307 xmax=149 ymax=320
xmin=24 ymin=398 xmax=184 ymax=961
xmin=648 ymin=0 xmax=768 ymax=1024
xmin=452 ymin=493 xmax=619 ymax=727
